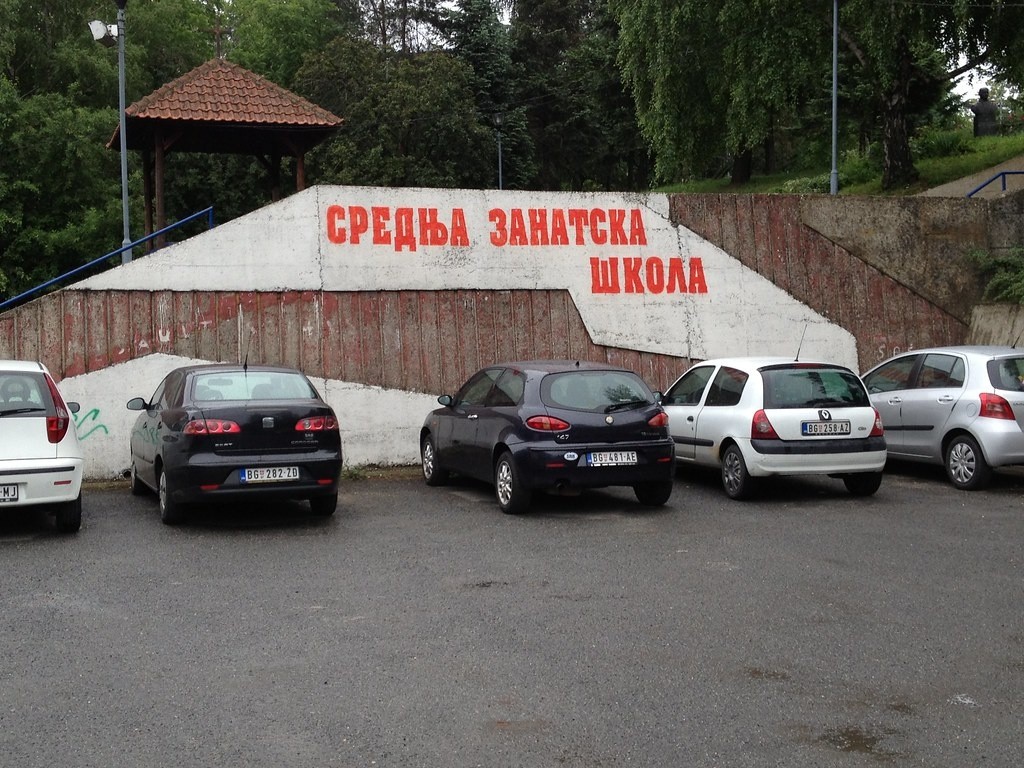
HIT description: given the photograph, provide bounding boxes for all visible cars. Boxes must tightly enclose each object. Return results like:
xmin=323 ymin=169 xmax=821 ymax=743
xmin=651 ymin=356 xmax=888 ymax=501
xmin=418 ymin=357 xmax=678 ymax=515
xmin=126 ymin=363 xmax=345 ymax=525
xmin=0 ymin=358 xmax=85 ymax=532
xmin=857 ymin=342 xmax=1024 ymax=491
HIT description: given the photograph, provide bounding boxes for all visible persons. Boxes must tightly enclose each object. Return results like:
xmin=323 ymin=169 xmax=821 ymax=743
xmin=970 ymin=87 xmax=1001 ymax=122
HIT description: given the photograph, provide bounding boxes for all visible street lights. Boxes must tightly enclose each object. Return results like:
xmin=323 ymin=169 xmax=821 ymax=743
xmin=478 ymin=108 xmax=522 ymax=190
xmin=87 ymin=1 xmax=133 ymax=265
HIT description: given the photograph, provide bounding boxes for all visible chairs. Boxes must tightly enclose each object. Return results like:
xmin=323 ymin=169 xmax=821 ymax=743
xmin=1 ymin=377 xmax=30 ymax=402
xmin=251 ymin=384 xmax=278 ymax=399
xmin=200 ymin=390 xmax=223 ymax=400
xmin=934 ymin=360 xmax=955 ymax=379
xmin=566 ymin=380 xmax=592 ymax=396
xmin=721 ymin=377 xmax=743 ymax=397
xmin=196 ymin=385 xmax=209 ymax=400
